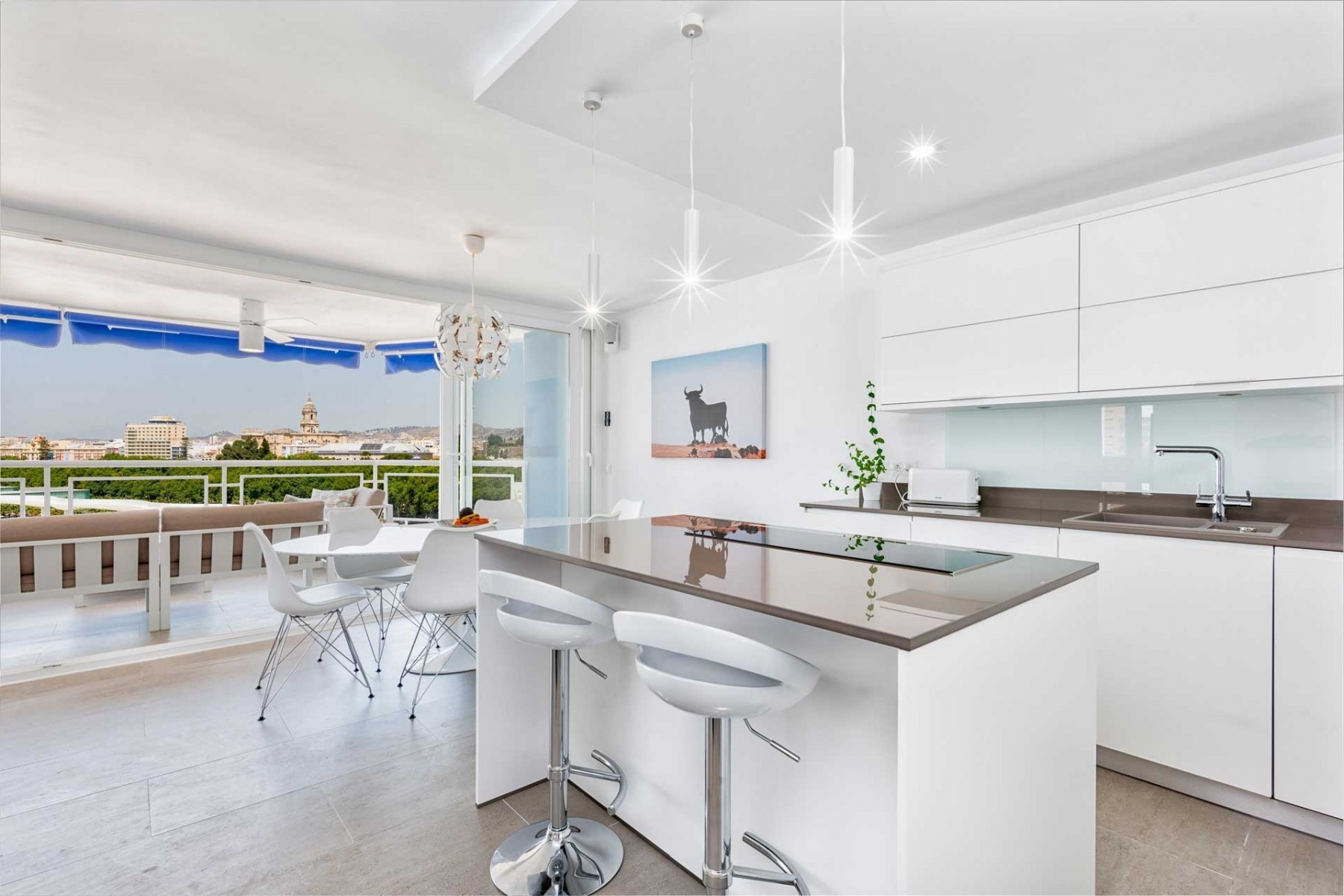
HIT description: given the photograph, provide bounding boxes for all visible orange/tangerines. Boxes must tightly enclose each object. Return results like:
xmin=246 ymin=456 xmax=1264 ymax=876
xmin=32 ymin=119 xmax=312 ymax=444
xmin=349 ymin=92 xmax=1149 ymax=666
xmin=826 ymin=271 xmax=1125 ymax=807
xmin=454 ymin=519 xmax=488 ymax=525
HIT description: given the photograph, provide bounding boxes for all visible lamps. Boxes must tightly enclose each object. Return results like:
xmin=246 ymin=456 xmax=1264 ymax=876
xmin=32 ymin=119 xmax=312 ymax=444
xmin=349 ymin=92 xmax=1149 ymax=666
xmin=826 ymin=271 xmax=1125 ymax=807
xmin=680 ymin=13 xmax=703 ymax=279
xmin=238 ymin=299 xmax=264 ymax=353
xmin=431 ymin=234 xmax=510 ymax=384
xmin=584 ymin=91 xmax=602 ymax=304
xmin=833 ymin=0 xmax=856 ymax=232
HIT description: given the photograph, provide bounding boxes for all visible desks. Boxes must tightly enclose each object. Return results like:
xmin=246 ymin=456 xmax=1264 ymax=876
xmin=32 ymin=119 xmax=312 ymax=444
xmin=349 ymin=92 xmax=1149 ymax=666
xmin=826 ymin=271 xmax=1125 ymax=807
xmin=475 ymin=515 xmax=1101 ymax=896
xmin=274 ymin=527 xmax=432 ymax=672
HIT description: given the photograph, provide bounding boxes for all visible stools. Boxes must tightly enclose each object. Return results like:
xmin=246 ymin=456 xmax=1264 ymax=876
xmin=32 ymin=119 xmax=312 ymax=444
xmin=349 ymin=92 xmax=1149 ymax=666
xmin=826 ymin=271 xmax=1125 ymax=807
xmin=612 ymin=611 xmax=819 ymax=896
xmin=480 ymin=569 xmax=624 ymax=896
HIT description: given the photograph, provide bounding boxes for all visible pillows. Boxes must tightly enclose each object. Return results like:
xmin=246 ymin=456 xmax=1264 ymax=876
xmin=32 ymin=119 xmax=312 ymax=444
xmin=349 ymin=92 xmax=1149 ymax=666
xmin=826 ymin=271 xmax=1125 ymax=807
xmin=284 ymin=494 xmax=311 ymax=502
xmin=311 ymin=488 xmax=358 ymax=520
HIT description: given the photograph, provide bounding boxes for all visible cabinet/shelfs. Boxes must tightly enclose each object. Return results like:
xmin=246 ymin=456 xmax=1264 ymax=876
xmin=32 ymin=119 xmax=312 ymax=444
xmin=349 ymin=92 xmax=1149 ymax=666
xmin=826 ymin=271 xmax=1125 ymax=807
xmin=807 ymin=506 xmax=1344 ymax=847
xmin=879 ymin=160 xmax=1344 ymax=406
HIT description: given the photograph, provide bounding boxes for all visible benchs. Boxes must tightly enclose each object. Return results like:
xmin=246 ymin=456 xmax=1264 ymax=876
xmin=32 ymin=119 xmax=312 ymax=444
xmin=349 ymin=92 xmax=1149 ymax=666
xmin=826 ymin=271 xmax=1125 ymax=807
xmin=323 ymin=487 xmax=393 ymax=524
xmin=0 ymin=508 xmax=161 ymax=632
xmin=161 ymin=502 xmax=328 ymax=630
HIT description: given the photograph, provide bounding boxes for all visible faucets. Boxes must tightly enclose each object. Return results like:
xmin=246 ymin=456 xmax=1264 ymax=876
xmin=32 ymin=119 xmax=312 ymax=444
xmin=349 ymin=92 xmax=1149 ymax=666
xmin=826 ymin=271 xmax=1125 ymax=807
xmin=1155 ymin=444 xmax=1255 ymax=522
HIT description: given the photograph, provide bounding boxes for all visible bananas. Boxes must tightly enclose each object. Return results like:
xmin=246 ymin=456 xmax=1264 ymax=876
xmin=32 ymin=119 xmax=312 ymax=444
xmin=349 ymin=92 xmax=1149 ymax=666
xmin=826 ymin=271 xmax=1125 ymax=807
xmin=461 ymin=513 xmax=480 ymax=523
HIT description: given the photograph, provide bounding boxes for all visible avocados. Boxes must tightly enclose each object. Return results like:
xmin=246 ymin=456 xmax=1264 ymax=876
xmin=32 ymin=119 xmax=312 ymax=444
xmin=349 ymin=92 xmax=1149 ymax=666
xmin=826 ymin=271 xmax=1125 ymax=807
xmin=459 ymin=508 xmax=473 ymax=517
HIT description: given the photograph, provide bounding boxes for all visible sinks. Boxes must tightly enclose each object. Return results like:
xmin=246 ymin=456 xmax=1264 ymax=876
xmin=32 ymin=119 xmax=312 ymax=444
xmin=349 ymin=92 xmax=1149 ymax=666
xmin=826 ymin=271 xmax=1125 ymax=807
xmin=1208 ymin=521 xmax=1279 ymax=534
xmin=1071 ymin=512 xmax=1211 ymax=528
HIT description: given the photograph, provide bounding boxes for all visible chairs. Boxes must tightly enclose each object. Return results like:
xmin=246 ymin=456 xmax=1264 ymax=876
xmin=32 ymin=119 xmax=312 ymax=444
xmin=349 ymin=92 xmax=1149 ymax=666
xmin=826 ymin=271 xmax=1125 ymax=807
xmin=244 ymin=500 xmax=649 ymax=721
